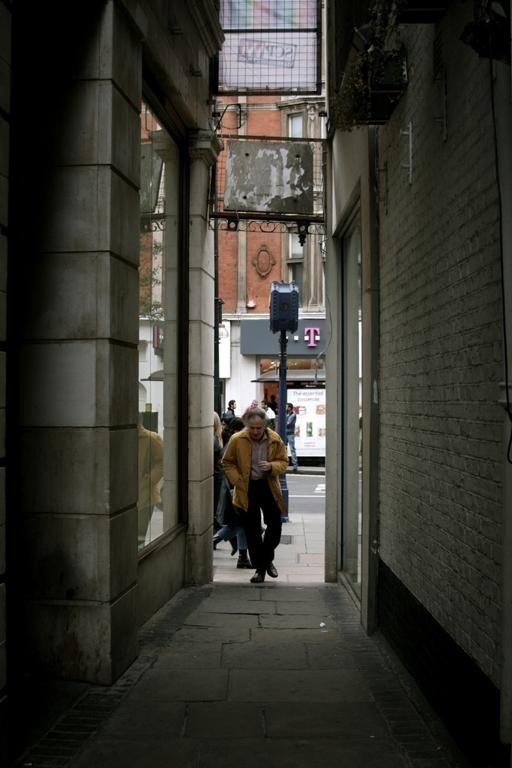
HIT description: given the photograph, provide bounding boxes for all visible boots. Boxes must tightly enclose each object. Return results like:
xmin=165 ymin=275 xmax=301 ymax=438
xmin=237 ymin=549 xmax=253 ymax=569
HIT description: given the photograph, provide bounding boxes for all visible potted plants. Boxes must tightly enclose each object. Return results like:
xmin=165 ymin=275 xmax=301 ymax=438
xmin=326 ymin=46 xmax=405 ymax=125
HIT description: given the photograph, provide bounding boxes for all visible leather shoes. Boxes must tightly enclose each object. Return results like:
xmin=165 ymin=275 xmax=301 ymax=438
xmin=250 ymin=560 xmax=278 ymax=583
xmin=212 ymin=533 xmax=238 ymax=556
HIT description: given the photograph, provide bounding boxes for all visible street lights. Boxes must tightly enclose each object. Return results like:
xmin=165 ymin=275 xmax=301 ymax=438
xmin=268 ymin=280 xmax=299 ymax=521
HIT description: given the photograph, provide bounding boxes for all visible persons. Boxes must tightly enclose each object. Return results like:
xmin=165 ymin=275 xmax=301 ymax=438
xmin=138 ymin=412 xmax=165 ymax=546
xmin=213 ymin=392 xmax=299 ymax=582
